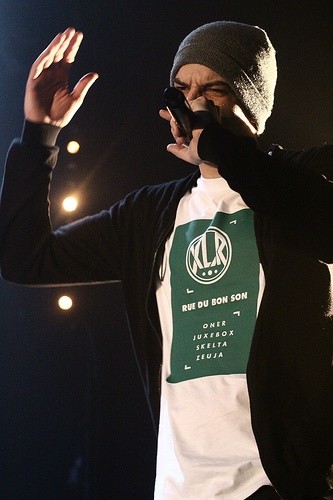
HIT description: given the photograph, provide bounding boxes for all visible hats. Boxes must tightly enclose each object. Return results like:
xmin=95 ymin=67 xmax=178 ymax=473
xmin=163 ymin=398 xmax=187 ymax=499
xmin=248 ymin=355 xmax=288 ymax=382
xmin=169 ymin=21 xmax=278 ymax=137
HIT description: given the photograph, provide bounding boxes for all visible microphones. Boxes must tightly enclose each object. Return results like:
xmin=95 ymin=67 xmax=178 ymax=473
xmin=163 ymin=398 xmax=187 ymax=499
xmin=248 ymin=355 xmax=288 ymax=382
xmin=164 ymin=87 xmax=194 ymax=148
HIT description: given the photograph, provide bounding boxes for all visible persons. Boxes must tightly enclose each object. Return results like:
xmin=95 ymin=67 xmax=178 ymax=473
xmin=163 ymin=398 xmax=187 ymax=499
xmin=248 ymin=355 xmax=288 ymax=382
xmin=0 ymin=20 xmax=332 ymax=500
